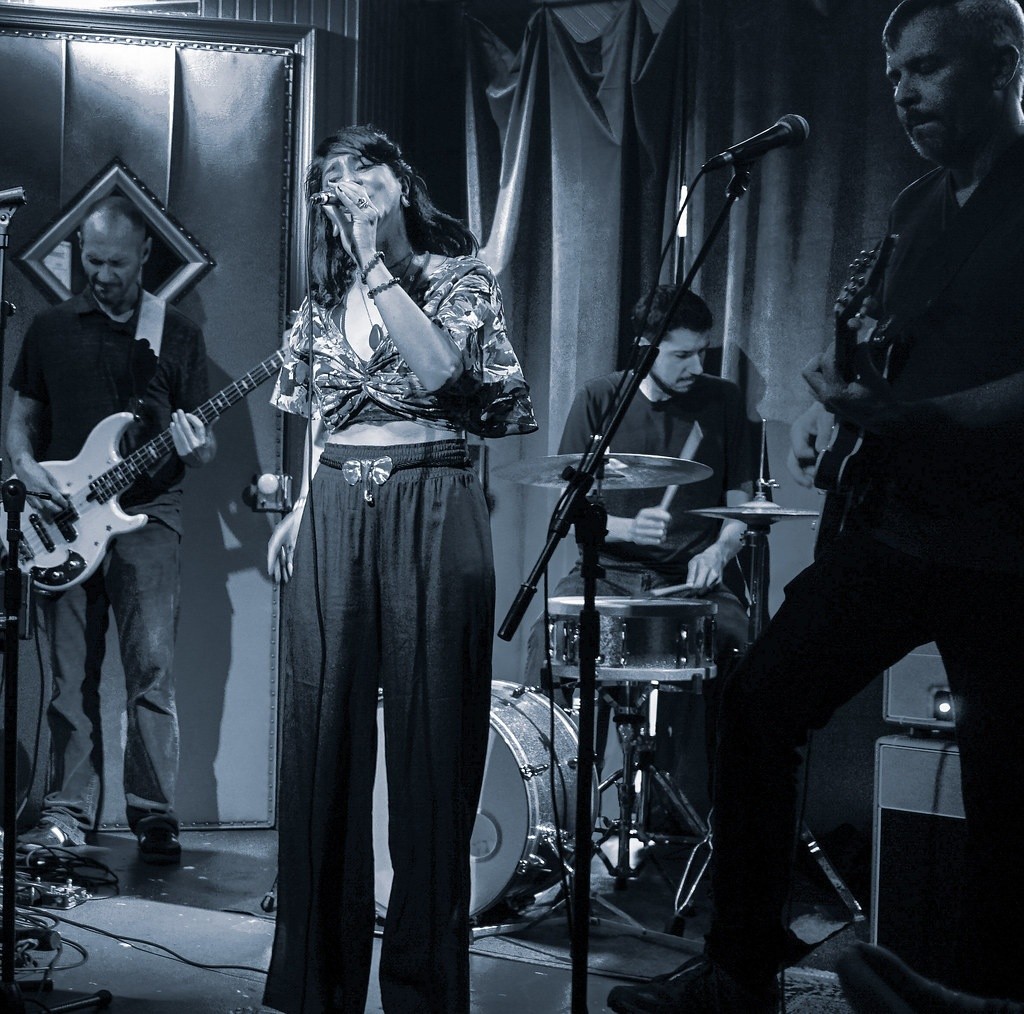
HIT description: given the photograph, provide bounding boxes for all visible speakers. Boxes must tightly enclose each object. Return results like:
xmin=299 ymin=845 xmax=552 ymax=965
xmin=867 ymin=734 xmax=992 ymax=1000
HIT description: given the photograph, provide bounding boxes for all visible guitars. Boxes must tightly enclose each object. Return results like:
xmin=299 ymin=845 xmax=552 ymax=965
xmin=0 ymin=344 xmax=292 ymax=600
xmin=811 ymin=242 xmax=902 ymax=566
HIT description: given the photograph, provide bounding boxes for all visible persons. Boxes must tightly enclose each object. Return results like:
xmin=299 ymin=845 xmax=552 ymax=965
xmin=605 ymin=0 xmax=1024 ymax=1014
xmin=260 ymin=119 xmax=539 ymax=1014
xmin=522 ymin=284 xmax=761 ymax=714
xmin=4 ymin=196 xmax=218 ymax=865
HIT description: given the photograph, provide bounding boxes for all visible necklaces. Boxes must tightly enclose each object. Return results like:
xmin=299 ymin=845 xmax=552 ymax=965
xmin=357 ymin=251 xmax=415 ymax=349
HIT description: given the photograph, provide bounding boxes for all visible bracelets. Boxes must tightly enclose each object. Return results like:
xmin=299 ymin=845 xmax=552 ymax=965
xmin=293 ymin=497 xmax=306 ymax=512
xmin=367 ymin=277 xmax=402 ymax=299
xmin=360 ymin=251 xmax=386 ymax=285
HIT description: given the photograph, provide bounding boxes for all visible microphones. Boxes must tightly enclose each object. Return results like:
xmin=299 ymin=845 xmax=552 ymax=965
xmin=310 ymin=191 xmax=345 ymax=205
xmin=702 ymin=114 xmax=810 ymax=173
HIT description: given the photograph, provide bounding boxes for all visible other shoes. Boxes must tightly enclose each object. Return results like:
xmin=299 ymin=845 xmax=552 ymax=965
xmin=228 ymin=1004 xmax=286 ymax=1014
xmin=18 ymin=817 xmax=85 ymax=847
xmin=138 ymin=822 xmax=181 ymax=863
xmin=607 ymin=956 xmax=779 ymax=1014
xmin=837 ymin=941 xmax=1024 ymax=1014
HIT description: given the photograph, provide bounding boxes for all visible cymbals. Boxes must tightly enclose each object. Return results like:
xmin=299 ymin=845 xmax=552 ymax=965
xmin=683 ymin=491 xmax=821 ymax=527
xmin=490 ymin=434 xmax=715 ymax=494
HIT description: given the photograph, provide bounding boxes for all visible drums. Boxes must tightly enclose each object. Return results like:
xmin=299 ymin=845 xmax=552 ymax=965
xmin=548 ymin=593 xmax=720 ymax=688
xmin=361 ymin=675 xmax=606 ymax=938
